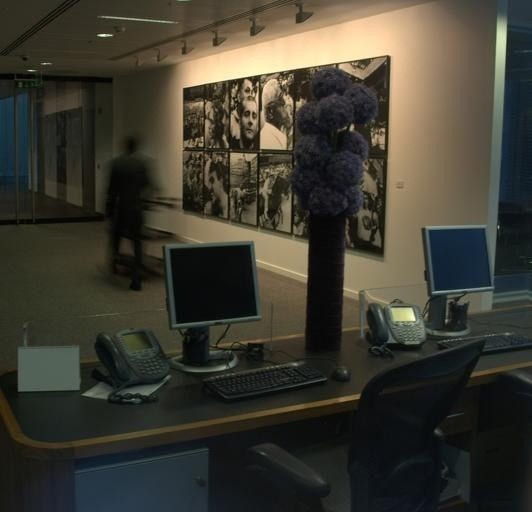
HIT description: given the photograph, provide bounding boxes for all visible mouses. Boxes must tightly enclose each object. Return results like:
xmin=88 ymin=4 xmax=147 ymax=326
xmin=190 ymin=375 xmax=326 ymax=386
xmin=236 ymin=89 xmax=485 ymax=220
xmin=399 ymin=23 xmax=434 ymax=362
xmin=332 ymin=365 xmax=352 ymax=385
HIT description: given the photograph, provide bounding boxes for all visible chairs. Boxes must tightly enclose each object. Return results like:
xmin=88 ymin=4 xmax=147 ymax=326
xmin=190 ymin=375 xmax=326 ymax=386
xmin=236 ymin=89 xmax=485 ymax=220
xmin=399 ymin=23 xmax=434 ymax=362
xmin=250 ymin=336 xmax=487 ymax=512
xmin=497 ymin=368 xmax=532 ymax=406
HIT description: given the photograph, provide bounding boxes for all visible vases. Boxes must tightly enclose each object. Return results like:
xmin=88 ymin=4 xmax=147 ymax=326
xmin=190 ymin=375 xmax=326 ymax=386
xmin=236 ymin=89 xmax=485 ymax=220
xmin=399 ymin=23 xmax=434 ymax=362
xmin=306 ymin=218 xmax=345 ymax=351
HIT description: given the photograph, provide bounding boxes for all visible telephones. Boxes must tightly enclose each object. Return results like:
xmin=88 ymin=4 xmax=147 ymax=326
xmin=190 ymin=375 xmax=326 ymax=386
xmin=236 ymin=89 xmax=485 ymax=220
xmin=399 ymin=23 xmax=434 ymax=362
xmin=366 ymin=302 xmax=427 ymax=351
xmin=94 ymin=327 xmax=171 ymax=386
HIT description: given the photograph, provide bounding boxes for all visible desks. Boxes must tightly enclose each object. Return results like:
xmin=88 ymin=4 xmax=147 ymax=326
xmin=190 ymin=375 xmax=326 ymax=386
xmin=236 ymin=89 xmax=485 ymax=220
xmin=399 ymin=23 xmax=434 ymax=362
xmin=1 ymin=306 xmax=532 ymax=512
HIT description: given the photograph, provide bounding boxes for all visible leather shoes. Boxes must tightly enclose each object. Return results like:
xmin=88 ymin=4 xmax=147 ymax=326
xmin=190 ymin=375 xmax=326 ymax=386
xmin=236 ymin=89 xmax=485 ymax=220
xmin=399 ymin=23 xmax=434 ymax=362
xmin=129 ymin=281 xmax=142 ymax=291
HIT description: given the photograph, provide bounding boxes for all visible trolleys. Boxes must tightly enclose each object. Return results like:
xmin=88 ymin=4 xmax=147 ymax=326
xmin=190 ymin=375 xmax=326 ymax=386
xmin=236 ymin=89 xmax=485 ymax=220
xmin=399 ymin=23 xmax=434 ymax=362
xmin=117 ymin=196 xmax=182 ymax=283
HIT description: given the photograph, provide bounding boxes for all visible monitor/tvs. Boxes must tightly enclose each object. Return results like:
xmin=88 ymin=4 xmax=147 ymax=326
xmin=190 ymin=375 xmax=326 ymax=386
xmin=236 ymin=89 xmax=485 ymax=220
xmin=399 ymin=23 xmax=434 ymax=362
xmin=162 ymin=241 xmax=263 ymax=375
xmin=418 ymin=223 xmax=496 ymax=337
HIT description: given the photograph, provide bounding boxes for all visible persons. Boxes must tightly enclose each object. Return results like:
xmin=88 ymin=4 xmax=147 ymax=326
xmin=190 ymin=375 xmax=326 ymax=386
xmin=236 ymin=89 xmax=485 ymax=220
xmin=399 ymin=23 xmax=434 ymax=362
xmin=182 ymin=67 xmax=385 ymax=248
xmin=103 ymin=136 xmax=146 ymax=292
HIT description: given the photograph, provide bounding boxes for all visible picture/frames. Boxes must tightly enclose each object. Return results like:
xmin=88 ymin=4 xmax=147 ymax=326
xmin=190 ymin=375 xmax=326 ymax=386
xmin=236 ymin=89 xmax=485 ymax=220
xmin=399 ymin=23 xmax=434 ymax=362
xmin=182 ymin=55 xmax=391 ymax=260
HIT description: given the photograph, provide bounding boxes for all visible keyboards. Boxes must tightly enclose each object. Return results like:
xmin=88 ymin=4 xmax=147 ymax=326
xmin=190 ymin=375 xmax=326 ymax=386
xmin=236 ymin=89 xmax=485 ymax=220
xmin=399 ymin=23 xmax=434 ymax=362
xmin=437 ymin=330 xmax=531 ymax=356
xmin=200 ymin=356 xmax=328 ymax=405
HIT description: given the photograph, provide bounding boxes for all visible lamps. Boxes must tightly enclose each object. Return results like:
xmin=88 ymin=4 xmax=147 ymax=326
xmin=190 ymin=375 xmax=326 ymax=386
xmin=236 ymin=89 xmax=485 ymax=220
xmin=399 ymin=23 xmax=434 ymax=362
xmin=135 ymin=1 xmax=314 ymax=69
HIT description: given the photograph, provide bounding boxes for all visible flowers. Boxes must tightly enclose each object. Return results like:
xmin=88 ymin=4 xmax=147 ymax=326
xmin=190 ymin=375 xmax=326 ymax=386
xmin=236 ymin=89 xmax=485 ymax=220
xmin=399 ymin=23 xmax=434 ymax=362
xmin=290 ymin=69 xmax=378 ymax=218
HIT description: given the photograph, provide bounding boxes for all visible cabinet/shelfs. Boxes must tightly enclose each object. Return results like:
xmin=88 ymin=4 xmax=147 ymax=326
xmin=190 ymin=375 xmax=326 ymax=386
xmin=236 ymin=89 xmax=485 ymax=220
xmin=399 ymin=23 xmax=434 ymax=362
xmin=74 ymin=448 xmax=209 ymax=512
